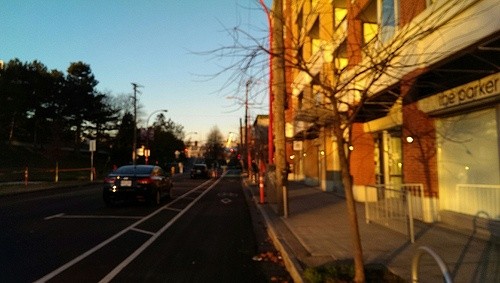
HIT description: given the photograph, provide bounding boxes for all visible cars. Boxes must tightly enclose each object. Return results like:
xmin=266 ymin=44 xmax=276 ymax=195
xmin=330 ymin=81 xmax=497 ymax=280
xmin=190 ymin=163 xmax=209 ymax=179
xmin=104 ymin=165 xmax=174 ymax=204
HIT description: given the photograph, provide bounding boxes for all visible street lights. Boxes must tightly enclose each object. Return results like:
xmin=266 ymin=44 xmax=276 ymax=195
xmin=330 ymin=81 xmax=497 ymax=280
xmin=146 ymin=109 xmax=167 ymax=164
